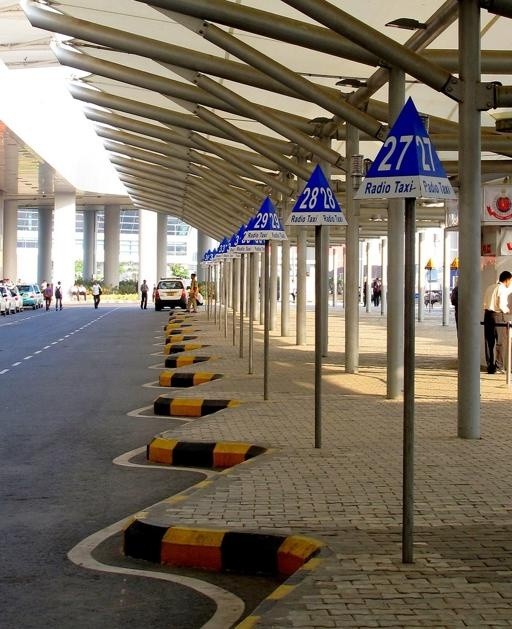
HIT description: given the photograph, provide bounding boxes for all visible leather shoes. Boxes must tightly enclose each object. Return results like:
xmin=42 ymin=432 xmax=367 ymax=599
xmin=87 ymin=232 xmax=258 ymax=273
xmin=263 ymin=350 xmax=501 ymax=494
xmin=487 ymin=369 xmax=503 ymax=374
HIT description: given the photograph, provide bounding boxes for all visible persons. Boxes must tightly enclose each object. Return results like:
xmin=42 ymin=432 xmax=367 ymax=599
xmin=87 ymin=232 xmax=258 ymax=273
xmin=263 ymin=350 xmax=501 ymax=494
xmin=152 ymin=273 xmax=204 ymax=313
xmin=362 ymin=278 xmax=381 ymax=307
xmin=140 ymin=280 xmax=149 ymax=310
xmin=91 ymin=281 xmax=102 ymax=308
xmin=424 ymin=289 xmax=440 ymax=307
xmin=0 ymin=276 xmax=87 ymax=311
xmin=290 ymin=280 xmax=296 ymax=303
xmin=450 ymin=283 xmax=459 ymax=338
xmin=482 ymin=270 xmax=512 ymax=374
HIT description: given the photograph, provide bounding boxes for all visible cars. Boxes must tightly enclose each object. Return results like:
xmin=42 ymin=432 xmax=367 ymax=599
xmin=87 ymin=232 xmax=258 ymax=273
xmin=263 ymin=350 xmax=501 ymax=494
xmin=0 ymin=279 xmax=43 ymax=317
xmin=154 ymin=276 xmax=188 ymax=311
